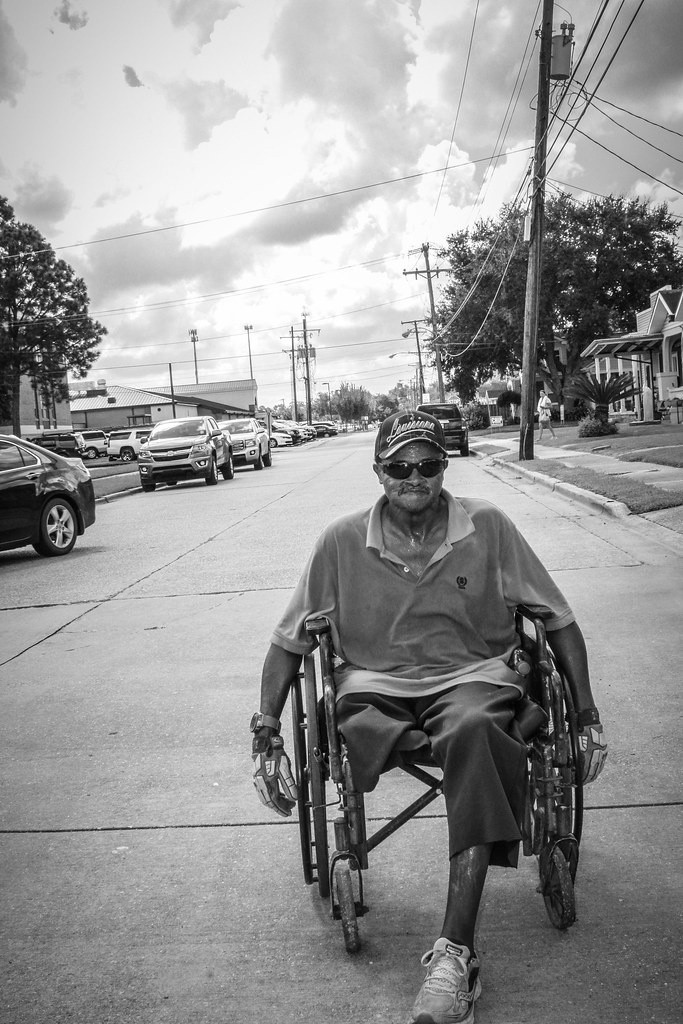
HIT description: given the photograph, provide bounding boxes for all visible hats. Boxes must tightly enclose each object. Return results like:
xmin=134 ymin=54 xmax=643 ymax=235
xmin=374 ymin=411 xmax=448 ymax=459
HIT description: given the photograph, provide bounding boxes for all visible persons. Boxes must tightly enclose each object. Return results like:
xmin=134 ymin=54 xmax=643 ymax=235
xmin=535 ymin=390 xmax=557 ymax=441
xmin=250 ymin=409 xmax=608 ymax=1022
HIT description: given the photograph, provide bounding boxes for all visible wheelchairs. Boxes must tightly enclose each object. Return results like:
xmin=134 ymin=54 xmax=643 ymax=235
xmin=287 ymin=602 xmax=584 ymax=951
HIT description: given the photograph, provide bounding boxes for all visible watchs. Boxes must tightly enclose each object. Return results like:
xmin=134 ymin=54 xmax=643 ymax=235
xmin=250 ymin=712 xmax=281 ymax=734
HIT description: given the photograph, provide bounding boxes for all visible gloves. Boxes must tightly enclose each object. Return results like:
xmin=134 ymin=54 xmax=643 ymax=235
xmin=251 ymin=736 xmax=298 ymax=817
xmin=565 ymin=707 xmax=607 ymax=785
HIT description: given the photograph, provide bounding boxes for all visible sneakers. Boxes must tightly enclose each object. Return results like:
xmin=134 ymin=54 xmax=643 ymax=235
xmin=407 ymin=937 xmax=481 ymax=1024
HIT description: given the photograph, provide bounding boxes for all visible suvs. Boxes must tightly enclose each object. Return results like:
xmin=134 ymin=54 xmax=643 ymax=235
xmin=25 ymin=432 xmax=89 ymax=457
xmin=105 ymin=427 xmax=154 ymax=462
xmin=69 ymin=428 xmax=109 ymax=460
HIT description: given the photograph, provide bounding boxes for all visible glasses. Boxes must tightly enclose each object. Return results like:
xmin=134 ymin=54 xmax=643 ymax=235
xmin=377 ymin=459 xmax=448 ymax=479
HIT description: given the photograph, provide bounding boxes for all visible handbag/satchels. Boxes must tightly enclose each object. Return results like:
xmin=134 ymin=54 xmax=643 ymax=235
xmin=539 ymin=407 xmax=550 ymax=423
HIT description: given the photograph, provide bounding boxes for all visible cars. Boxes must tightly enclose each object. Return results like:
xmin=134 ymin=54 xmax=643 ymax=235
xmin=137 ymin=414 xmax=234 ymax=492
xmin=415 ymin=401 xmax=473 ymax=457
xmin=217 ymin=418 xmax=272 ymax=470
xmin=0 ymin=433 xmax=96 ymax=558
xmin=258 ymin=418 xmax=339 ymax=448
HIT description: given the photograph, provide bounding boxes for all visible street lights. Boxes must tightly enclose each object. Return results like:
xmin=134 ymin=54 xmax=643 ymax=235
xmin=401 ymin=327 xmax=447 ymax=403
xmin=388 ymin=350 xmax=427 ymax=394
xmin=322 ymin=382 xmax=332 ymax=421
xmin=244 ymin=324 xmax=254 ymax=378
xmin=188 ymin=328 xmax=199 ymax=384
